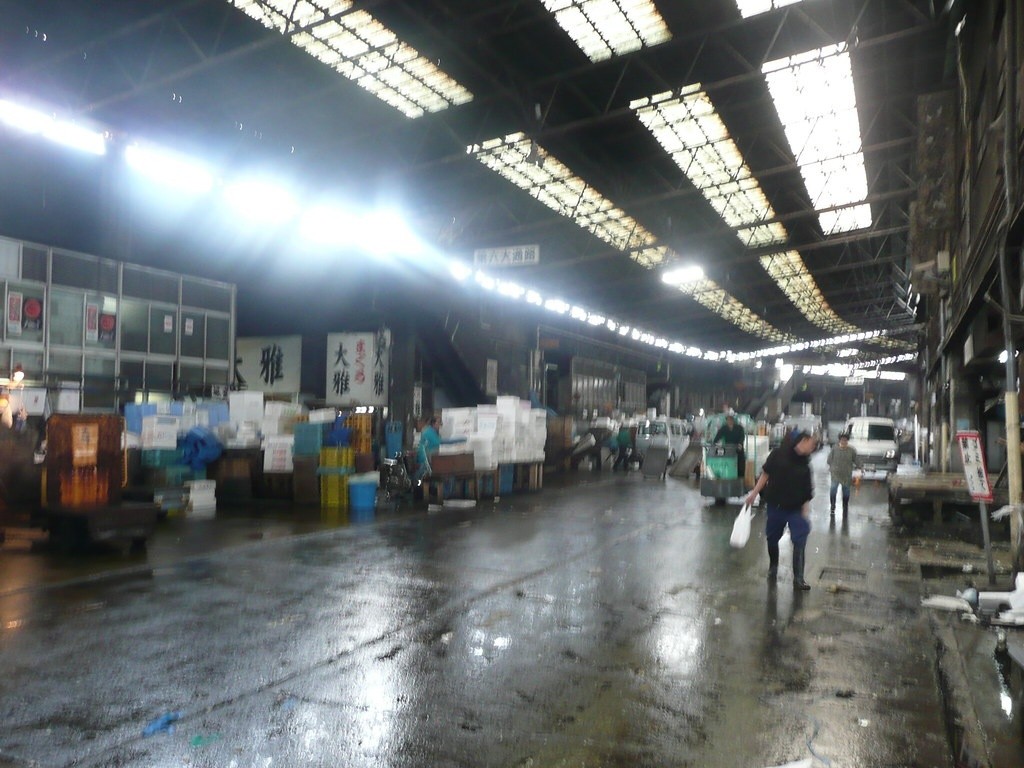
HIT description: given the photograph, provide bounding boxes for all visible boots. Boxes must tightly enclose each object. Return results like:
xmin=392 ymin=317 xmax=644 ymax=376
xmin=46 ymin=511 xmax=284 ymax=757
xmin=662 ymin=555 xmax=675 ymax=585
xmin=789 ymin=553 xmax=812 ymax=591
xmin=766 ymin=546 xmax=780 ymax=578
xmin=843 ymin=496 xmax=849 ymax=516
xmin=829 ymin=497 xmax=837 ymax=512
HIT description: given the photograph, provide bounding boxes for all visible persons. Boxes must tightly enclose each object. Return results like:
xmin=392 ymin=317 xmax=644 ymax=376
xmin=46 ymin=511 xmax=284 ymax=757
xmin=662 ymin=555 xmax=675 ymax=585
xmin=418 ymin=416 xmax=441 ymax=450
xmin=712 ymin=414 xmax=746 ymax=479
xmin=745 ymin=428 xmax=816 ymax=592
xmin=826 ymin=429 xmax=864 ymax=523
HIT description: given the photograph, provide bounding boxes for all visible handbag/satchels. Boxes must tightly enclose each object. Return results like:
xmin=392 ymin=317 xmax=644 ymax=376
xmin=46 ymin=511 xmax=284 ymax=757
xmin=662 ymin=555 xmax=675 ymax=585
xmin=729 ymin=502 xmax=755 ymax=549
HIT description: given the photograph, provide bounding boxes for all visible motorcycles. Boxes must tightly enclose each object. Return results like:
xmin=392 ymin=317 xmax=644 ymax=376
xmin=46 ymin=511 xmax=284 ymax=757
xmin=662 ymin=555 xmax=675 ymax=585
xmin=701 ymin=412 xmax=765 ymax=506
xmin=373 ymin=449 xmax=419 ymax=511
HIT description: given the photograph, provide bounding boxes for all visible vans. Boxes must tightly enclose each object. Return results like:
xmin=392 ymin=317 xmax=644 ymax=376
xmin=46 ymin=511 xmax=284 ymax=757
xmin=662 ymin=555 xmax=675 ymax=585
xmin=842 ymin=417 xmax=898 ymax=475
xmin=636 ymin=418 xmax=692 ymax=464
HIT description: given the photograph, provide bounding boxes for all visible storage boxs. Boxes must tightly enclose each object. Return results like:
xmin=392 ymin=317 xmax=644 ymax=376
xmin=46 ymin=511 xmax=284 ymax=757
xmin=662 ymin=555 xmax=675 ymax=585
xmin=121 ymin=388 xmax=547 ymax=512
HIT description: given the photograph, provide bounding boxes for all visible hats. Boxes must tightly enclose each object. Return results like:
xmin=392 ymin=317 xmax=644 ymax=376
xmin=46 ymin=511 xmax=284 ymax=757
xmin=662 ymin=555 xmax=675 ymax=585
xmin=837 ymin=431 xmax=850 ymax=441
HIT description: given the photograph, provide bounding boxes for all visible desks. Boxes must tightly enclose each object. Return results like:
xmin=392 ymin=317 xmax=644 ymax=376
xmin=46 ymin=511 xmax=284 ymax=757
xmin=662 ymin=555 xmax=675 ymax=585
xmin=420 ymin=470 xmax=499 ymax=505
